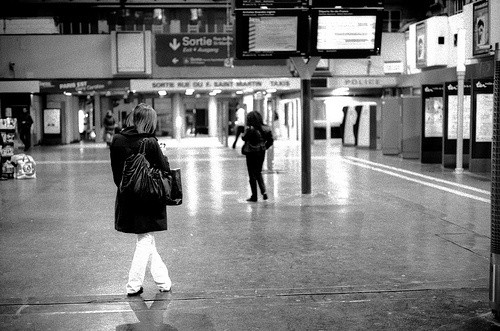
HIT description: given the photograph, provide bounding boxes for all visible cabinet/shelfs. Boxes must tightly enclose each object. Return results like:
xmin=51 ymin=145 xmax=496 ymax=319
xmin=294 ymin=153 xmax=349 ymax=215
xmin=0 ymin=122 xmax=26 ymax=178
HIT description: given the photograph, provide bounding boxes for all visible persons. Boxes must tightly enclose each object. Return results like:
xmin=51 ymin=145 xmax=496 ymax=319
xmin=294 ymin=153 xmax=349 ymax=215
xmin=103 ymin=110 xmax=115 ymax=145
xmin=15 ymin=108 xmax=34 ymax=151
xmin=110 ymin=103 xmax=172 ymax=296
xmin=232 ymin=105 xmax=274 ymax=203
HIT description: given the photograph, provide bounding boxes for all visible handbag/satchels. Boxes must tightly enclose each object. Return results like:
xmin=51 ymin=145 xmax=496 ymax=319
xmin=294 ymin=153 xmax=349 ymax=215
xmin=16 ymin=155 xmax=36 ymax=179
xmin=241 ymin=130 xmax=266 ymax=154
xmin=119 ymin=139 xmax=182 ymax=206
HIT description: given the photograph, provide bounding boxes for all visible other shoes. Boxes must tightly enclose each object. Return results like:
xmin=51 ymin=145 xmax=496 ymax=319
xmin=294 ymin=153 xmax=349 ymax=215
xmin=160 ymin=288 xmax=171 ymax=293
xmin=127 ymin=287 xmax=143 ymax=297
xmin=246 ymin=197 xmax=257 ymax=202
xmin=263 ymin=194 xmax=267 ymax=200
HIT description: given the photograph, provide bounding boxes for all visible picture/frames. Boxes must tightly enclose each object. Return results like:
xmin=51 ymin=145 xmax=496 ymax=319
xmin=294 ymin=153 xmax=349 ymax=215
xmin=472 ymin=0 xmax=491 ymax=56
xmin=416 ymin=21 xmax=427 ymax=67
